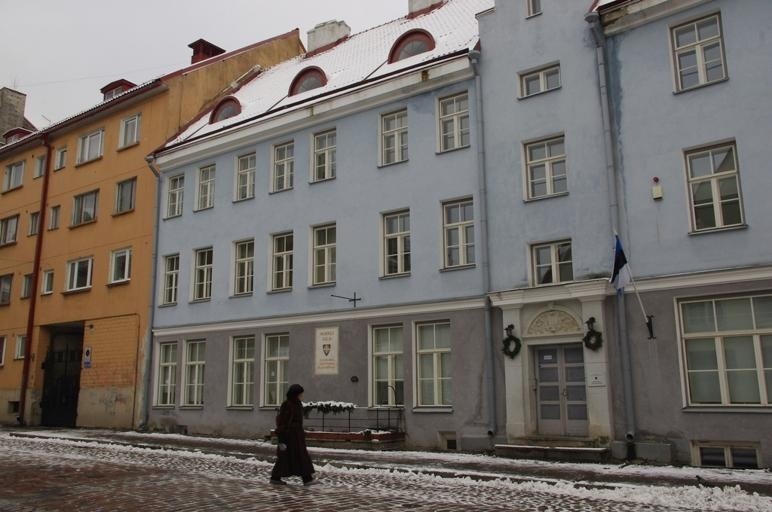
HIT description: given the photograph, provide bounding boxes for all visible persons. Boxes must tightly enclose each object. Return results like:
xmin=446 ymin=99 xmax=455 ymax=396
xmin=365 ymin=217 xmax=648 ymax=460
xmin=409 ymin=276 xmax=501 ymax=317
xmin=270 ymin=383 xmax=319 ymax=486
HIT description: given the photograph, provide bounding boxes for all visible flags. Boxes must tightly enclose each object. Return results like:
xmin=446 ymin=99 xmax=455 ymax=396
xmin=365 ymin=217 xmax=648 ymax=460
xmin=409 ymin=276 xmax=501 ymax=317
xmin=608 ymin=238 xmax=629 ymax=301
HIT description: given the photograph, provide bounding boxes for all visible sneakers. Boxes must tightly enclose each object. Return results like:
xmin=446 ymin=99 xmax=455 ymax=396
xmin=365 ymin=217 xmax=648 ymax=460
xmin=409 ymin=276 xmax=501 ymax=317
xmin=303 ymin=478 xmax=319 ymax=487
xmin=269 ymin=477 xmax=287 ymax=485
